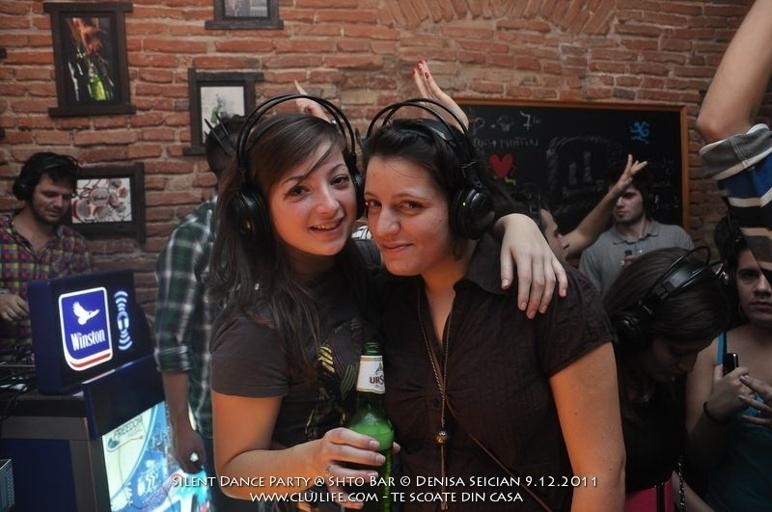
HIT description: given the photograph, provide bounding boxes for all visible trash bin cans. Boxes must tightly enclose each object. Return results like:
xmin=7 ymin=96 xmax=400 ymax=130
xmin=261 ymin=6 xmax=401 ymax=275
xmin=0 ymin=458 xmax=15 ymax=511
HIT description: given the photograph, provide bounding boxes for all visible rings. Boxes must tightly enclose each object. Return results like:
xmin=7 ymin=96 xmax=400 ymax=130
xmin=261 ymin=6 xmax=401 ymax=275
xmin=325 ymin=465 xmax=336 ymax=479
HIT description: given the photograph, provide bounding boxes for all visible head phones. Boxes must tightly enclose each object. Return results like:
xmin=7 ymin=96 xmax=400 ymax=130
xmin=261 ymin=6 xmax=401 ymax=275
xmin=615 ymin=244 xmax=727 ymax=359
xmin=12 ymin=155 xmax=78 ymax=200
xmin=604 ymin=159 xmax=664 ymax=217
xmin=367 ymin=96 xmax=497 ymax=242
xmin=231 ymin=93 xmax=365 ymax=249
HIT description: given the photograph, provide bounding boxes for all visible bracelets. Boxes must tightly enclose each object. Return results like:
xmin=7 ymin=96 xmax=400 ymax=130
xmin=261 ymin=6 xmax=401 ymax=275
xmin=701 ymin=400 xmax=730 ymax=425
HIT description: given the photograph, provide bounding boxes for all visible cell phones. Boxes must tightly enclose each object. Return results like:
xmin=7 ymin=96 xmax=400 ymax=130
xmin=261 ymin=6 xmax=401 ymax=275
xmin=722 ymin=352 xmax=739 ymax=375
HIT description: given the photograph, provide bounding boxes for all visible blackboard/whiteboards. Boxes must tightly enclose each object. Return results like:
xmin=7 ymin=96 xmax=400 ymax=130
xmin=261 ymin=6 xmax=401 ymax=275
xmin=450 ymin=98 xmax=689 ymax=267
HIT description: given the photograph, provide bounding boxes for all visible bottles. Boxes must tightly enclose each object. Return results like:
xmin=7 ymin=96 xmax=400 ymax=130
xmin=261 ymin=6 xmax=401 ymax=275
xmin=341 ymin=339 xmax=395 ymax=512
xmin=303 ymin=346 xmax=351 ymax=512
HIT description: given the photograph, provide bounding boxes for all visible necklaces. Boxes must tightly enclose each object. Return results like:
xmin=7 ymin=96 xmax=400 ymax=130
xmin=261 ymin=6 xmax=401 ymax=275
xmin=416 ymin=278 xmax=455 ymax=511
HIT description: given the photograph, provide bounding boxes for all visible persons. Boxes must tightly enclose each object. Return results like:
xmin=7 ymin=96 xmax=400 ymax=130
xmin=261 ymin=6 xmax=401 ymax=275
xmin=0 ymin=149 xmax=98 ymax=378
xmin=209 ymin=112 xmax=567 ymax=511
xmin=504 ymin=147 xmax=649 ymax=264
xmin=682 ymin=208 xmax=772 ymax=512
xmin=410 ymin=57 xmax=470 ymax=135
xmin=574 ymin=165 xmax=696 ymax=298
xmin=151 ymin=112 xmax=259 ymax=511
xmin=693 ymin=1 xmax=771 ymax=282
xmin=602 ymin=245 xmax=730 ymax=511
xmin=292 ymin=79 xmax=388 ymax=127
xmin=296 ymin=120 xmax=628 ymax=512
